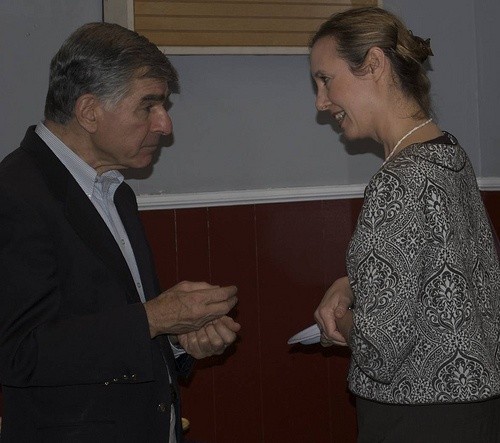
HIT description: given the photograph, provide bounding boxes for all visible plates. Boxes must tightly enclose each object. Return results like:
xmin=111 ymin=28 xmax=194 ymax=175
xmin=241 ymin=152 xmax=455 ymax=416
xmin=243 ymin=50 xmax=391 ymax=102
xmin=287 ymin=322 xmax=322 ymax=345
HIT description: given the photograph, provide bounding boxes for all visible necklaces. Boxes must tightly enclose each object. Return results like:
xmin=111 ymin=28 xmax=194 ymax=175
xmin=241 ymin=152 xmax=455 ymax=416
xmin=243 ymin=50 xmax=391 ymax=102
xmin=379 ymin=119 xmax=433 ymax=167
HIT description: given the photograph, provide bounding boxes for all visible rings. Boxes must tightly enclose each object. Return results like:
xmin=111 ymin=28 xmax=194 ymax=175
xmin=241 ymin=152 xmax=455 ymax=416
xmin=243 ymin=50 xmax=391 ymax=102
xmin=320 ymin=329 xmax=323 ymax=334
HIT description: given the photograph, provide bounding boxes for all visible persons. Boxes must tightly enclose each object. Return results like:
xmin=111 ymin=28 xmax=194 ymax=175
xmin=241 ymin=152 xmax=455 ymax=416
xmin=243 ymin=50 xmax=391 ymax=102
xmin=309 ymin=7 xmax=500 ymax=443
xmin=1 ymin=21 xmax=242 ymax=442
